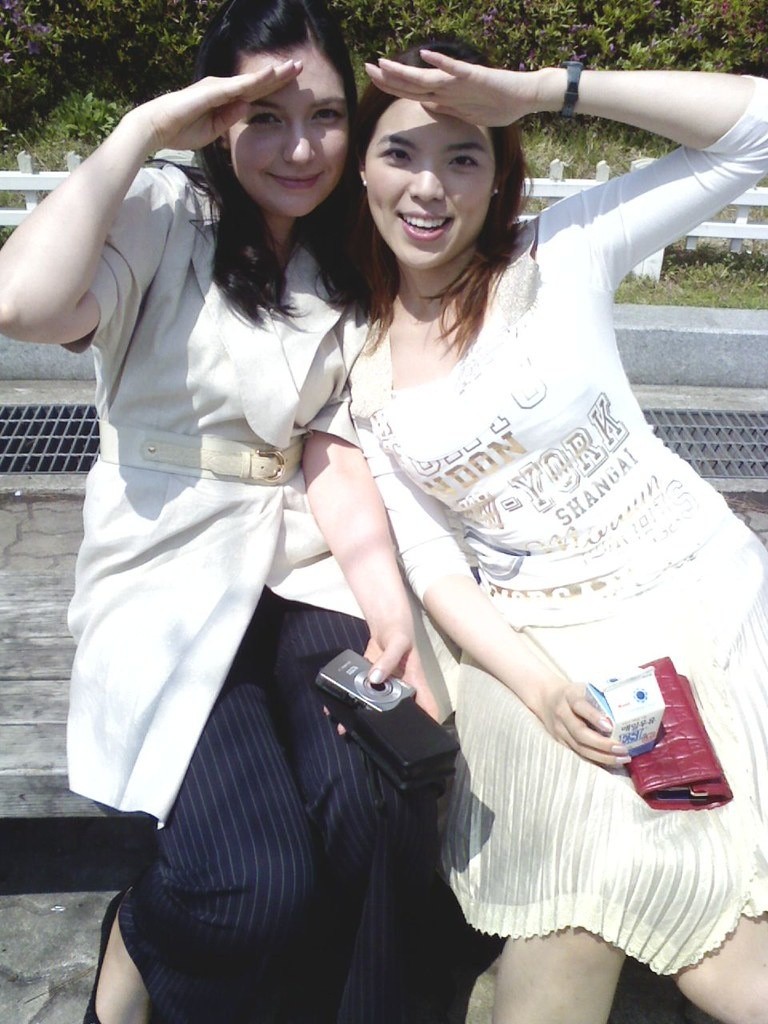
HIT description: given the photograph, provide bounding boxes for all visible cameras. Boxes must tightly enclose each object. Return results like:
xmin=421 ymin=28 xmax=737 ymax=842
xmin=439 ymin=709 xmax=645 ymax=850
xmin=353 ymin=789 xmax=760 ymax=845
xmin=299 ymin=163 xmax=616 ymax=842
xmin=315 ymin=649 xmax=417 ymax=713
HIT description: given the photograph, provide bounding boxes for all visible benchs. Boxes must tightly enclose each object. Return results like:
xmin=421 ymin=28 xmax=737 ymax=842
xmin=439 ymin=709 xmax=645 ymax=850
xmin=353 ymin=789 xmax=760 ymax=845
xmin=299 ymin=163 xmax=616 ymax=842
xmin=0 ymin=596 xmax=146 ymax=819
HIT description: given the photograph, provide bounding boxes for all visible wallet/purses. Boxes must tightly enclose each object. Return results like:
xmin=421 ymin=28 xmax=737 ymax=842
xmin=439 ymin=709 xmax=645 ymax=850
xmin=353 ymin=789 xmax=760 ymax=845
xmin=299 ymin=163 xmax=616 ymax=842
xmin=623 ymin=657 xmax=733 ymax=811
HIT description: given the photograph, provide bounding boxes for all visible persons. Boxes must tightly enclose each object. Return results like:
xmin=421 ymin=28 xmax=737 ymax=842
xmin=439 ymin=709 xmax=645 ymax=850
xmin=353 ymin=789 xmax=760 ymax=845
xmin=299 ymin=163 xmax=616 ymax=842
xmin=0 ymin=0 xmax=467 ymax=1023
xmin=313 ymin=41 xmax=768 ymax=1023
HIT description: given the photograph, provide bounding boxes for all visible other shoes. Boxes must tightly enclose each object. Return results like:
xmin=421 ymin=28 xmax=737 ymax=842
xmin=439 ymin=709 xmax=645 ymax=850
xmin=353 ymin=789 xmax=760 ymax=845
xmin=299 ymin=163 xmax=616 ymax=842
xmin=84 ymin=885 xmax=132 ymax=1024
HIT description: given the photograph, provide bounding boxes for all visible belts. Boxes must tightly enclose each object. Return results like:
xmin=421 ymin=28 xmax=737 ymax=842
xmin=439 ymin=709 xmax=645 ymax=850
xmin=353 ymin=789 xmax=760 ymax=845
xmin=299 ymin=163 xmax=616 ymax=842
xmin=99 ymin=422 xmax=303 ymax=485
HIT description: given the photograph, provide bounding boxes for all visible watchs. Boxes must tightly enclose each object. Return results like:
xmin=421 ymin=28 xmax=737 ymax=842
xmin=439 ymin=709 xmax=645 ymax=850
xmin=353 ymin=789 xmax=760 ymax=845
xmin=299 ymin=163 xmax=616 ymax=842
xmin=557 ymin=61 xmax=584 ymax=117
